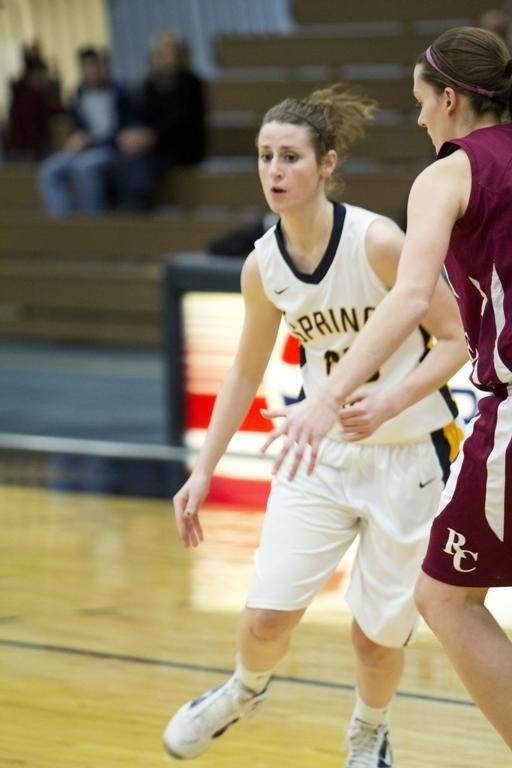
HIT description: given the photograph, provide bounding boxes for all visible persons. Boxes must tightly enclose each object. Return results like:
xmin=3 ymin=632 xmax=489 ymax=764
xmin=258 ymin=28 xmax=512 ymax=768
xmin=164 ymin=97 xmax=444 ymax=768
xmin=5 ymin=33 xmax=206 ymax=215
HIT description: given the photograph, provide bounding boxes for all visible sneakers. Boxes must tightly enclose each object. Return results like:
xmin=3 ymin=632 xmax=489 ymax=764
xmin=162 ymin=674 xmax=274 ymax=760
xmin=342 ymin=714 xmax=396 ymax=768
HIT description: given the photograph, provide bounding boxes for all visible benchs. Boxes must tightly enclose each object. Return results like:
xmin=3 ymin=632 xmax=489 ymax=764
xmin=0 ymin=2 xmax=511 ymax=349
